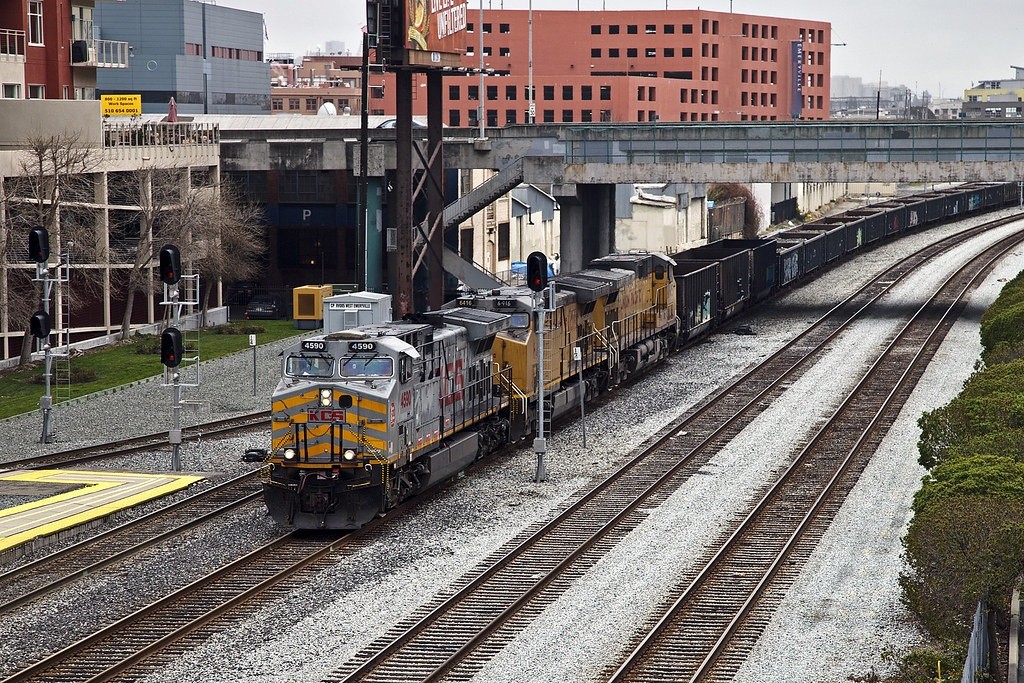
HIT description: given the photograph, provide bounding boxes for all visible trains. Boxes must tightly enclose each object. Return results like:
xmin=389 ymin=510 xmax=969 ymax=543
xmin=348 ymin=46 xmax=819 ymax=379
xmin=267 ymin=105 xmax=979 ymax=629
xmin=260 ymin=181 xmax=1021 ymax=531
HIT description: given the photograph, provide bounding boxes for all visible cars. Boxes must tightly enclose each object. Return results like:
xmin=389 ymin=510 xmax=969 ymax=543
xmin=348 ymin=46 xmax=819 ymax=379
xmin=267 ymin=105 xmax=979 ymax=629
xmin=368 ymin=117 xmax=455 ymax=142
xmin=243 ymin=293 xmax=289 ymax=320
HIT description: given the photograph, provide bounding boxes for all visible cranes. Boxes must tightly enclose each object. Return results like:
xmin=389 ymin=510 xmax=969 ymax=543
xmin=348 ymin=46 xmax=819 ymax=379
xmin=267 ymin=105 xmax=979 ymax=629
xmin=831 ymin=28 xmax=846 ymax=47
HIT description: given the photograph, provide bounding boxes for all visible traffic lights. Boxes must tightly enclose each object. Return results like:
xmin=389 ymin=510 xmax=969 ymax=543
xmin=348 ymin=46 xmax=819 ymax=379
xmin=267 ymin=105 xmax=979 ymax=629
xmin=527 ymin=251 xmax=547 ymax=291
xmin=161 ymin=327 xmax=183 ymax=367
xmin=28 ymin=225 xmax=49 ymax=263
xmin=160 ymin=243 xmax=181 ymax=285
xmin=30 ymin=312 xmax=51 ymax=339
